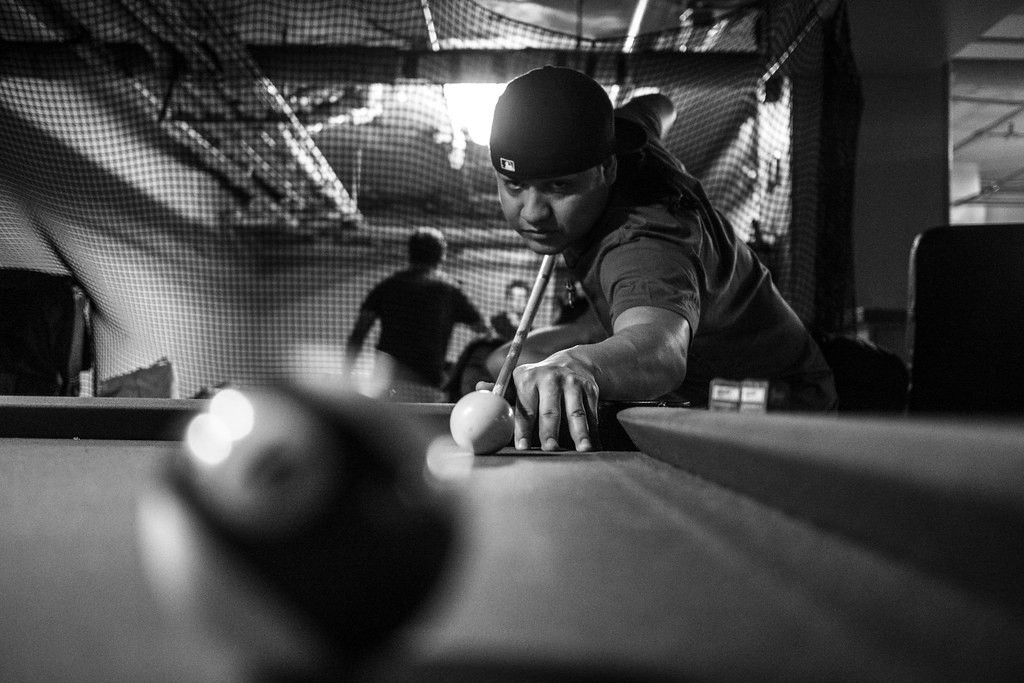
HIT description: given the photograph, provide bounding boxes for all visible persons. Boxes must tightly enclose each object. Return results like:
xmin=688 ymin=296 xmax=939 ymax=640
xmin=476 ymin=68 xmax=839 ymax=453
xmin=344 ymin=227 xmax=491 ymax=404
xmin=487 ymin=280 xmax=536 ymax=341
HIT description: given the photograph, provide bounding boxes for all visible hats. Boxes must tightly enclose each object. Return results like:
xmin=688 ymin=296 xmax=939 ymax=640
xmin=490 ymin=64 xmax=614 ymax=179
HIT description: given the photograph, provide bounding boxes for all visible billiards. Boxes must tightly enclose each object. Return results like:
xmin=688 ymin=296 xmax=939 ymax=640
xmin=137 ymin=373 xmax=475 ymax=683
xmin=449 ymin=390 xmax=513 ymax=454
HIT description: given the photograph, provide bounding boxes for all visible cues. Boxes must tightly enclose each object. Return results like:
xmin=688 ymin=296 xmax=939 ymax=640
xmin=491 ymin=253 xmax=557 ymax=398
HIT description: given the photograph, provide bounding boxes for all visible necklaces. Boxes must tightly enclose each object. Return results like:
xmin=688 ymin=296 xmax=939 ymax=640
xmin=562 ymin=198 xmax=613 ymax=310
